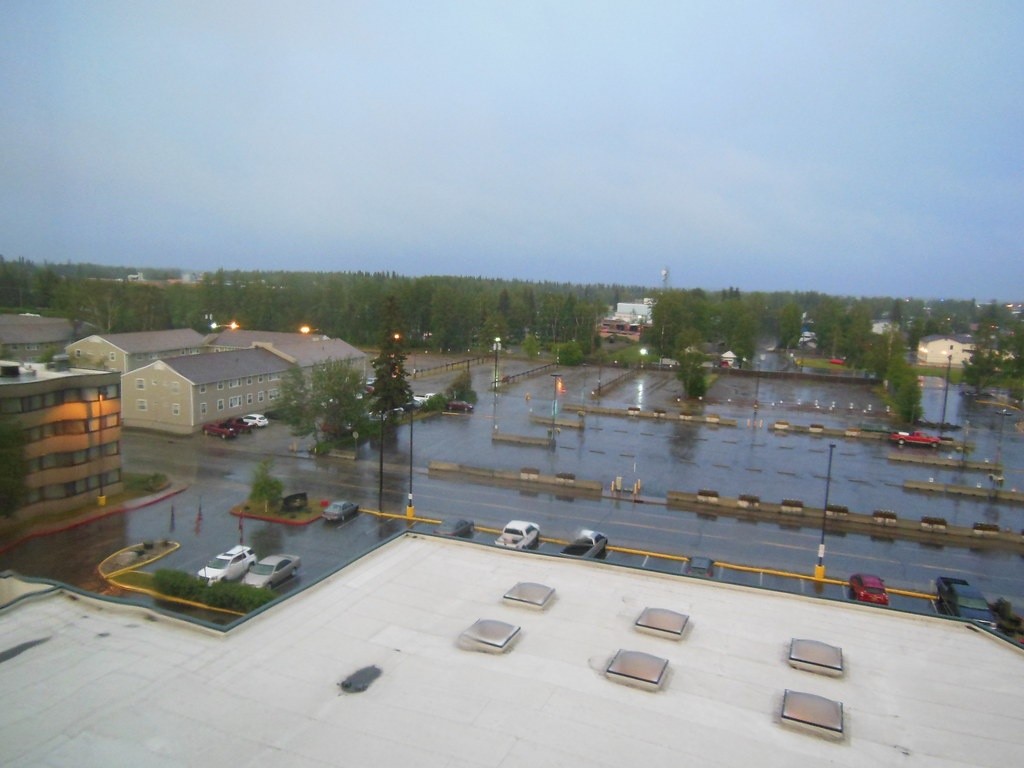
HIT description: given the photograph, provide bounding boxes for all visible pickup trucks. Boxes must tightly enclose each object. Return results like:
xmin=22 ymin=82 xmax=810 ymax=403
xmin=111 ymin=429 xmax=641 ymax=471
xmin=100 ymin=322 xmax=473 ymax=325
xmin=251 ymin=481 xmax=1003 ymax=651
xmin=495 ymin=521 xmax=541 ymax=551
xmin=226 ymin=416 xmax=253 ymax=433
xmin=937 ymin=577 xmax=999 ymax=633
xmin=561 ymin=530 xmax=610 ymax=558
xmin=204 ymin=423 xmax=236 ymax=439
xmin=892 ymin=430 xmax=944 ymax=450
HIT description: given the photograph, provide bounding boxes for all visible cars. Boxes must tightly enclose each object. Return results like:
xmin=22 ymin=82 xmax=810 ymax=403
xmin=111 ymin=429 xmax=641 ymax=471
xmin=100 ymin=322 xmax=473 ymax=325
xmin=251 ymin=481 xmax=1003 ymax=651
xmin=323 ymin=501 xmax=360 ymax=521
xmin=319 ymin=376 xmax=380 ymax=408
xmin=446 ymin=400 xmax=473 ymax=412
xmin=242 ymin=414 xmax=269 ymax=428
xmin=851 ymin=573 xmax=890 ymax=605
xmin=240 ymin=554 xmax=302 ymax=591
xmin=400 ymin=392 xmax=437 ymax=410
xmin=265 ymin=410 xmax=289 ymax=421
xmin=435 ymin=518 xmax=475 ymax=538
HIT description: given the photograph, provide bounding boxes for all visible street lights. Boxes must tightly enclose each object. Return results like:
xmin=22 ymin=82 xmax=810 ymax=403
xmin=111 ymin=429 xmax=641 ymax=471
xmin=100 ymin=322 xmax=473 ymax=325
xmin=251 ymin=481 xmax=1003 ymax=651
xmin=940 ymin=346 xmax=956 ymax=430
xmin=813 ymin=444 xmax=835 ymax=584
xmin=379 ymin=408 xmax=405 ymax=497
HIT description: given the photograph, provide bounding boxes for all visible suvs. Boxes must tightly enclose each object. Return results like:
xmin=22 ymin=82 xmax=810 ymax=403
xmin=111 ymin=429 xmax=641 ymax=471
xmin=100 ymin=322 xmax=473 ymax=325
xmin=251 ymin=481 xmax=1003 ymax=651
xmin=197 ymin=546 xmax=257 ymax=587
